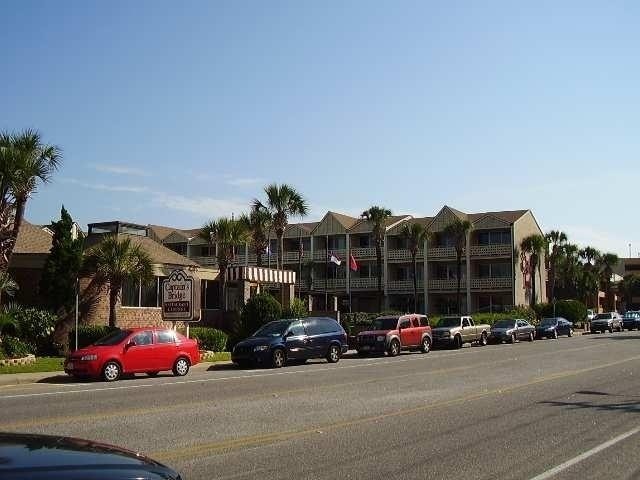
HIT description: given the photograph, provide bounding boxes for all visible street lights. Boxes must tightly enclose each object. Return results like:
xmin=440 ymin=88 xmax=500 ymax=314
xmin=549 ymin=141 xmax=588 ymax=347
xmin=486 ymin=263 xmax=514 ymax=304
xmin=628 ymin=243 xmax=634 ymax=274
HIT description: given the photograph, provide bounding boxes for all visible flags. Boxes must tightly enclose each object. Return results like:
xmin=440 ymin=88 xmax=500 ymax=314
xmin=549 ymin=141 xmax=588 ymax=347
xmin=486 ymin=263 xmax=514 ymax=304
xmin=330 ymin=250 xmax=341 ymax=266
xmin=351 ymin=256 xmax=359 ymax=272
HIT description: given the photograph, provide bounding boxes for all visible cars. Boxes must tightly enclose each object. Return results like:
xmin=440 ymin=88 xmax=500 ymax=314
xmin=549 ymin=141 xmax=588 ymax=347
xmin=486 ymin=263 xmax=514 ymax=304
xmin=0 ymin=427 xmax=183 ymax=480
xmin=60 ymin=324 xmax=206 ymax=384
xmin=487 ymin=306 xmax=640 ymax=345
xmin=228 ymin=315 xmax=352 ymax=372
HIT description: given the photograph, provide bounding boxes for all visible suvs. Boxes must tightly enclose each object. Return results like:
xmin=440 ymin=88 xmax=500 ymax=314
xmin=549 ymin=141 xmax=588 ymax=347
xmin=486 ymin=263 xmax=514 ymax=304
xmin=353 ymin=312 xmax=434 ymax=359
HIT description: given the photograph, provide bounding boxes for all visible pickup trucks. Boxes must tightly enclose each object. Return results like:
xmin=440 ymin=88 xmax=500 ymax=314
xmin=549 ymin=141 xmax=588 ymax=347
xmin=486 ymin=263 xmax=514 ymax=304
xmin=431 ymin=315 xmax=493 ymax=349
